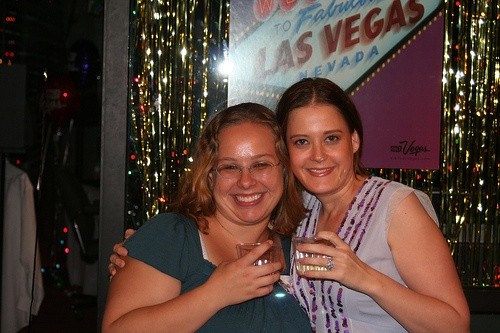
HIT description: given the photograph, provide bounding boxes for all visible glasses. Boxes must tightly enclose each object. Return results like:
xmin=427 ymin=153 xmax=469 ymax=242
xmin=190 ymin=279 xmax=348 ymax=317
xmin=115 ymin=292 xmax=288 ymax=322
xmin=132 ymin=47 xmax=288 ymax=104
xmin=212 ymin=160 xmax=282 ymax=178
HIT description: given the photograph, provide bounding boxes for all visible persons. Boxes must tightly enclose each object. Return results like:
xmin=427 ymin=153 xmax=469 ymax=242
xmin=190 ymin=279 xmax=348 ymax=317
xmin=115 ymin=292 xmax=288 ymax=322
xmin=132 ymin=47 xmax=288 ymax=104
xmin=62 ymin=166 xmax=100 ymax=296
xmin=108 ymin=76 xmax=470 ymax=333
xmin=102 ymin=102 xmax=314 ymax=333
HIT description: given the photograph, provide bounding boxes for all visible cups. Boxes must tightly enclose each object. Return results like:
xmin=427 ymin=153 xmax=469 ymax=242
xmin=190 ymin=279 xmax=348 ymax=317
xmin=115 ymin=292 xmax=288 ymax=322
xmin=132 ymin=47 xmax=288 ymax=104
xmin=235 ymin=243 xmax=279 ymax=276
xmin=292 ymin=237 xmax=336 ymax=276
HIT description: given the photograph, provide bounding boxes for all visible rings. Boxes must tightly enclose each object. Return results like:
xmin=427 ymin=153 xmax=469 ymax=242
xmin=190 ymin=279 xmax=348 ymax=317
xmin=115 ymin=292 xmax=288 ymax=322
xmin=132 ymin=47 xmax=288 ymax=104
xmin=326 ymin=257 xmax=333 ymax=269
